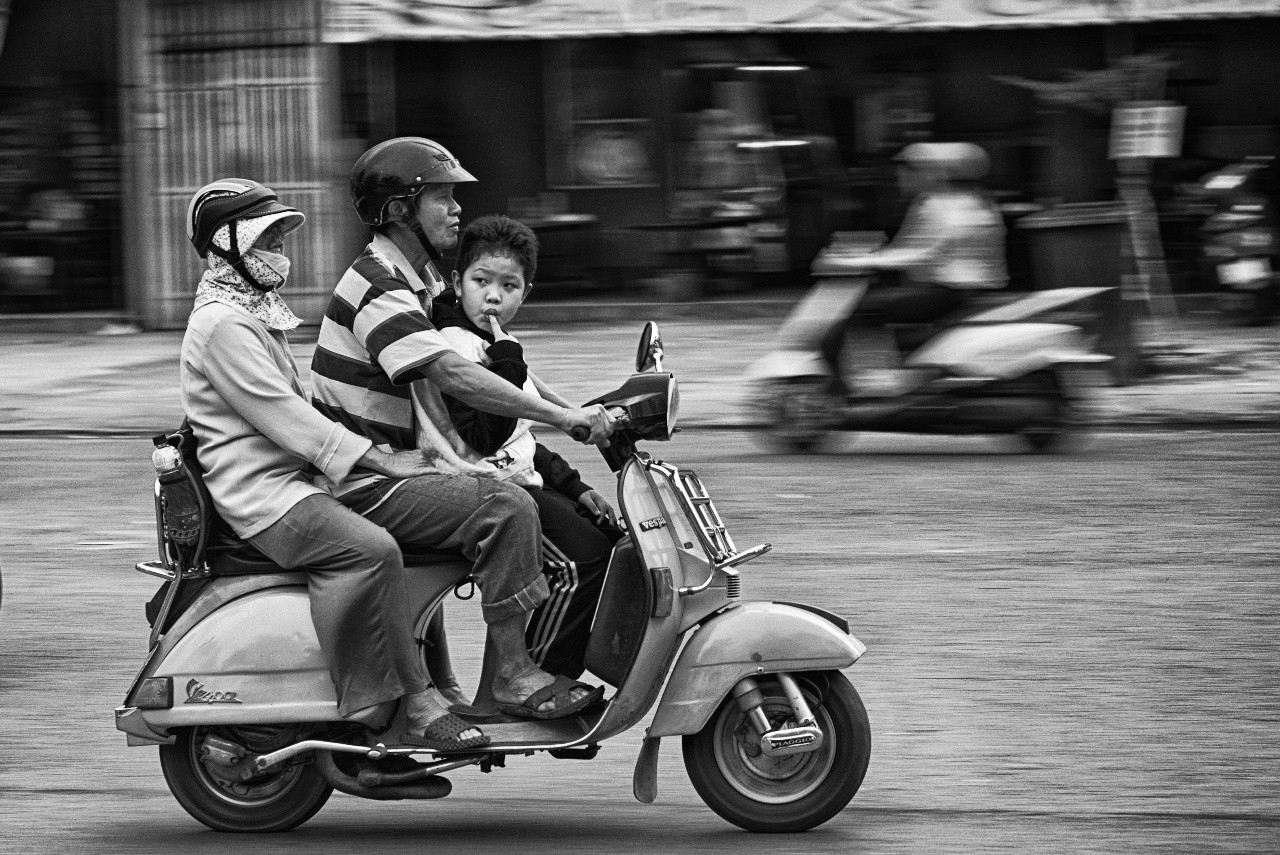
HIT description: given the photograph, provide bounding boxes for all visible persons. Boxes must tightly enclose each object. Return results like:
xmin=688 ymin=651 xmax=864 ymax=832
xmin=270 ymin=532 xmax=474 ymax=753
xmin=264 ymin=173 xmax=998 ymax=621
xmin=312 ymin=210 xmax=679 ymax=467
xmin=809 ymin=142 xmax=1009 ymax=397
xmin=849 ymin=53 xmax=913 ymax=289
xmin=181 ymin=178 xmax=491 ymax=757
xmin=310 ymin=137 xmax=616 ymax=721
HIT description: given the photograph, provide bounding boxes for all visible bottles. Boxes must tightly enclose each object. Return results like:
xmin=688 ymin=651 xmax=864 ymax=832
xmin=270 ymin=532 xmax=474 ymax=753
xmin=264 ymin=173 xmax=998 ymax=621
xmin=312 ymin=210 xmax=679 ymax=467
xmin=151 ymin=431 xmax=183 ymax=476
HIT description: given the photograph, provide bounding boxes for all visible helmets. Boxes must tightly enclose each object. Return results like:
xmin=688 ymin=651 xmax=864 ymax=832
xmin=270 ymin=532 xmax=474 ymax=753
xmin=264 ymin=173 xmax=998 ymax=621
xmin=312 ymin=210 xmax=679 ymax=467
xmin=896 ymin=141 xmax=988 ymax=184
xmin=186 ymin=178 xmax=298 ymax=259
xmin=349 ymin=137 xmax=479 ymax=226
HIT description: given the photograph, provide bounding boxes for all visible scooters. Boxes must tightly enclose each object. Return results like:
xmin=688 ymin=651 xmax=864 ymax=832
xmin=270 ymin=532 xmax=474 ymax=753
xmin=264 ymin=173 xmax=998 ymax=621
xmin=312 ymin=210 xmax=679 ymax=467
xmin=731 ymin=228 xmax=1125 ymax=452
xmin=113 ymin=316 xmax=874 ymax=839
xmin=1174 ymin=153 xmax=1280 ymax=314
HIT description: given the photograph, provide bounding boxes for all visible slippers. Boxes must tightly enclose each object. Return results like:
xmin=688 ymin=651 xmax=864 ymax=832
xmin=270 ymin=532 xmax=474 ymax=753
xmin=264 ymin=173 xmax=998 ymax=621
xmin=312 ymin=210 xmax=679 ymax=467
xmin=406 ymin=711 xmax=490 ymax=750
xmin=495 ymin=674 xmax=605 ymax=719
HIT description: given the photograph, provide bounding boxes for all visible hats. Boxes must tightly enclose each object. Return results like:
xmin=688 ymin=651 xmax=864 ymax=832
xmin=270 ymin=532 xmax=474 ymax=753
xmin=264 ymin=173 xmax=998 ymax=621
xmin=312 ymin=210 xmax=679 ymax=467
xmin=207 ymin=210 xmax=307 ymax=269
